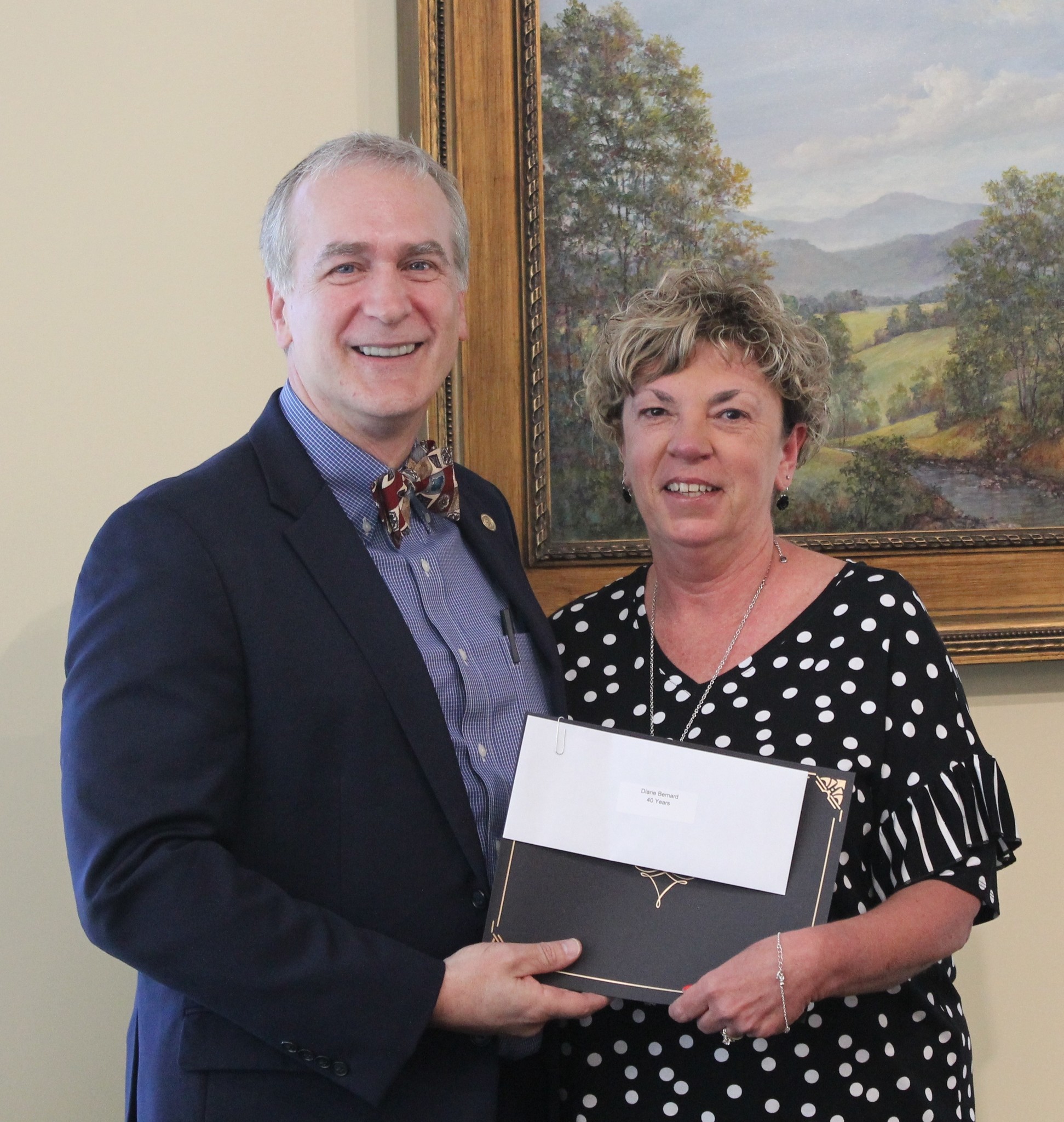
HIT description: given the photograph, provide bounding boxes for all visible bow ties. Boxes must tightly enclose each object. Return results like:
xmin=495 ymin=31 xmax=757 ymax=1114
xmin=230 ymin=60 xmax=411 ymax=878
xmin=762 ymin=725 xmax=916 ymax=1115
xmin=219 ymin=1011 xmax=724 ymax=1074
xmin=371 ymin=441 xmax=461 ymax=552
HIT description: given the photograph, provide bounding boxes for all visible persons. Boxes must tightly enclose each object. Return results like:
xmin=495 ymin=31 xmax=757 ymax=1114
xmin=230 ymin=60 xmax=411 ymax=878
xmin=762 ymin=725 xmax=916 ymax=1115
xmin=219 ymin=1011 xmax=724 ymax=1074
xmin=541 ymin=262 xmax=1021 ymax=1122
xmin=60 ymin=129 xmax=610 ymax=1122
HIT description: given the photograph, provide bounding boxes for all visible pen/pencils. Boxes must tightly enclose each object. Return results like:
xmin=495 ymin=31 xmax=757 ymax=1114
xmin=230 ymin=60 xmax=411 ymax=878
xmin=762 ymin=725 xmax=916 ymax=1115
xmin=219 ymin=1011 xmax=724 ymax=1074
xmin=500 ymin=606 xmax=521 ymax=668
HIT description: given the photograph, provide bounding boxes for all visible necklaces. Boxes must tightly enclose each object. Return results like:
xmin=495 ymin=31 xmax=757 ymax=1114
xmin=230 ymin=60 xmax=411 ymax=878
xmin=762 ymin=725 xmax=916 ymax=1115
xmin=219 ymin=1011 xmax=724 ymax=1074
xmin=644 ymin=536 xmax=792 ymax=745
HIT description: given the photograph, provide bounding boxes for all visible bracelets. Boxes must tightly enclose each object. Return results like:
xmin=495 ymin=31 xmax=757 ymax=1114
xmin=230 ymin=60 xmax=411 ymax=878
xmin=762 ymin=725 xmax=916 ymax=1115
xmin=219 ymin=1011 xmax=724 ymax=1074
xmin=774 ymin=931 xmax=793 ymax=1035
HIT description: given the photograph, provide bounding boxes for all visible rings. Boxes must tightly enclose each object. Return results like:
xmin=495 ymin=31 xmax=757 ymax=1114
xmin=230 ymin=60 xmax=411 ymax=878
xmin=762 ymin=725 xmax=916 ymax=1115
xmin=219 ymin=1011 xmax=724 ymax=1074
xmin=720 ymin=1026 xmax=742 ymax=1045
